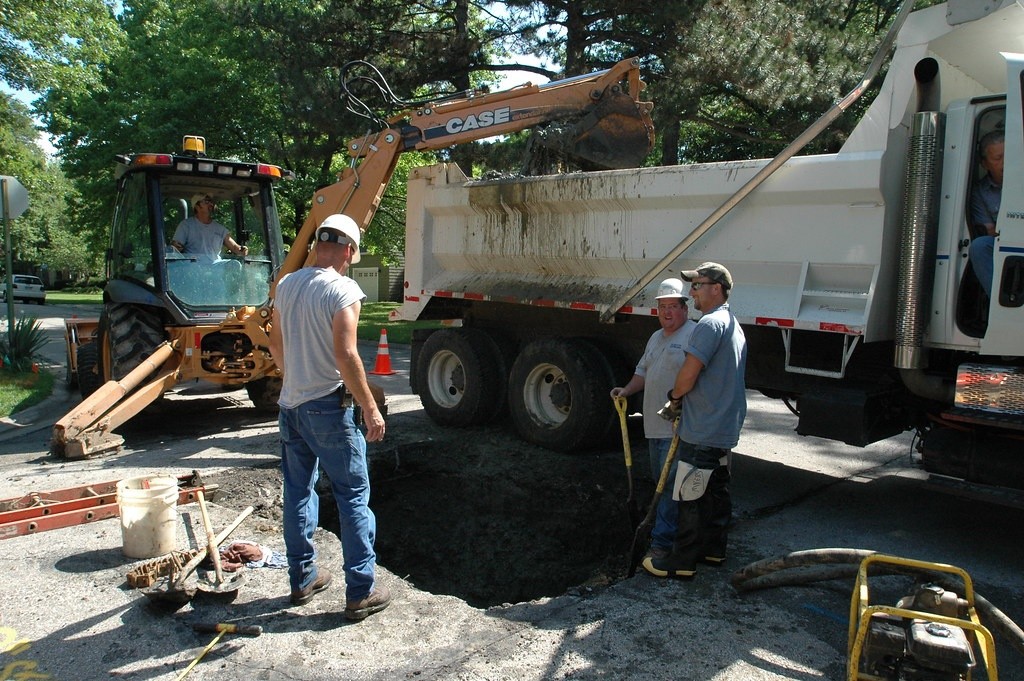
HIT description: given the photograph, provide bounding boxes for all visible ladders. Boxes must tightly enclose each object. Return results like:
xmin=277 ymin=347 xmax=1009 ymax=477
xmin=0 ymin=468 xmax=219 ymax=541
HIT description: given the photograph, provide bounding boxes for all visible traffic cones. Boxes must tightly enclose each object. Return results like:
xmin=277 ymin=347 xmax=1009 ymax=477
xmin=370 ymin=329 xmax=398 ymax=378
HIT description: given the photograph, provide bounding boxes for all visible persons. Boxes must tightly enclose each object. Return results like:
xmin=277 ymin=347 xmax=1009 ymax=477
xmin=170 ymin=192 xmax=249 ymax=304
xmin=268 ymin=214 xmax=390 ymax=620
xmin=610 ymin=279 xmax=700 ymax=564
xmin=638 ymin=263 xmax=747 ymax=579
xmin=969 ymin=130 xmax=1006 ymax=300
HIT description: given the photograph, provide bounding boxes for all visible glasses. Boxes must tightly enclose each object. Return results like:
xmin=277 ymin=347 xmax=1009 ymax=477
xmin=197 ymin=198 xmax=212 ymax=206
xmin=691 ymin=282 xmax=718 ymax=290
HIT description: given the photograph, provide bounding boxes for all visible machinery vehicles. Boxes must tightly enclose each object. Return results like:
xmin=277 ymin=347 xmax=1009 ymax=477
xmin=47 ymin=59 xmax=658 ymax=471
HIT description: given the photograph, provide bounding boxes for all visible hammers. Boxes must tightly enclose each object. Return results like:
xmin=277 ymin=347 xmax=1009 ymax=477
xmin=172 ymin=623 xmax=262 ymax=681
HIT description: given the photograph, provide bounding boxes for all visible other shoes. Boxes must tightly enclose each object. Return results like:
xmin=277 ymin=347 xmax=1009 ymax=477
xmin=700 ymin=555 xmax=726 ymax=563
xmin=642 ymin=556 xmax=696 ymax=576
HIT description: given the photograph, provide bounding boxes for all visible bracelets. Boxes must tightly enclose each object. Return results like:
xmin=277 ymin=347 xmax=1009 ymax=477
xmin=667 ymin=389 xmax=683 ymax=402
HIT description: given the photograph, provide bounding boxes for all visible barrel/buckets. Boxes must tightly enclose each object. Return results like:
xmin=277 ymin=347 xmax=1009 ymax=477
xmin=115 ymin=474 xmax=179 ymax=559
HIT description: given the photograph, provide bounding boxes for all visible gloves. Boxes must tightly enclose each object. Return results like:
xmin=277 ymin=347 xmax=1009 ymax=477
xmin=668 ymin=389 xmax=682 ymax=415
xmin=658 ymin=403 xmax=674 ymax=421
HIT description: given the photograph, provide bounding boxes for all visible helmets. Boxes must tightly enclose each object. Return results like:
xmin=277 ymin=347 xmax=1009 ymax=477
xmin=315 ymin=214 xmax=361 ymax=264
xmin=655 ymin=278 xmax=690 ymax=301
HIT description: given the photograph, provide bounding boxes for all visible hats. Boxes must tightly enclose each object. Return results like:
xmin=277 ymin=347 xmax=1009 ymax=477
xmin=191 ymin=192 xmax=214 ymax=211
xmin=681 ymin=262 xmax=732 ymax=289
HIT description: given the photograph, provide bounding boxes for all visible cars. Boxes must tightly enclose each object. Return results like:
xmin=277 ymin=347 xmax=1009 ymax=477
xmin=0 ymin=274 xmax=46 ymax=305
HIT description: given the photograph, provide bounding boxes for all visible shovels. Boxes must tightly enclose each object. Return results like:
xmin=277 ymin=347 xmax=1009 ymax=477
xmin=140 ymin=505 xmax=254 ymax=602
xmin=613 ymin=395 xmax=638 ymax=534
xmin=627 ymin=416 xmax=682 ymax=566
xmin=195 ymin=490 xmax=247 ymax=593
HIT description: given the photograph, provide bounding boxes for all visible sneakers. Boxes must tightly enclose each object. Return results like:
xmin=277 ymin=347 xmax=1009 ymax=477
xmin=344 ymin=588 xmax=390 ymax=620
xmin=291 ymin=569 xmax=331 ymax=605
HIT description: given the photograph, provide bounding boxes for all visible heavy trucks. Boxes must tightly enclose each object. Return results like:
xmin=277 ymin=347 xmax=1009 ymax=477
xmin=389 ymin=1 xmax=1024 ymax=529
